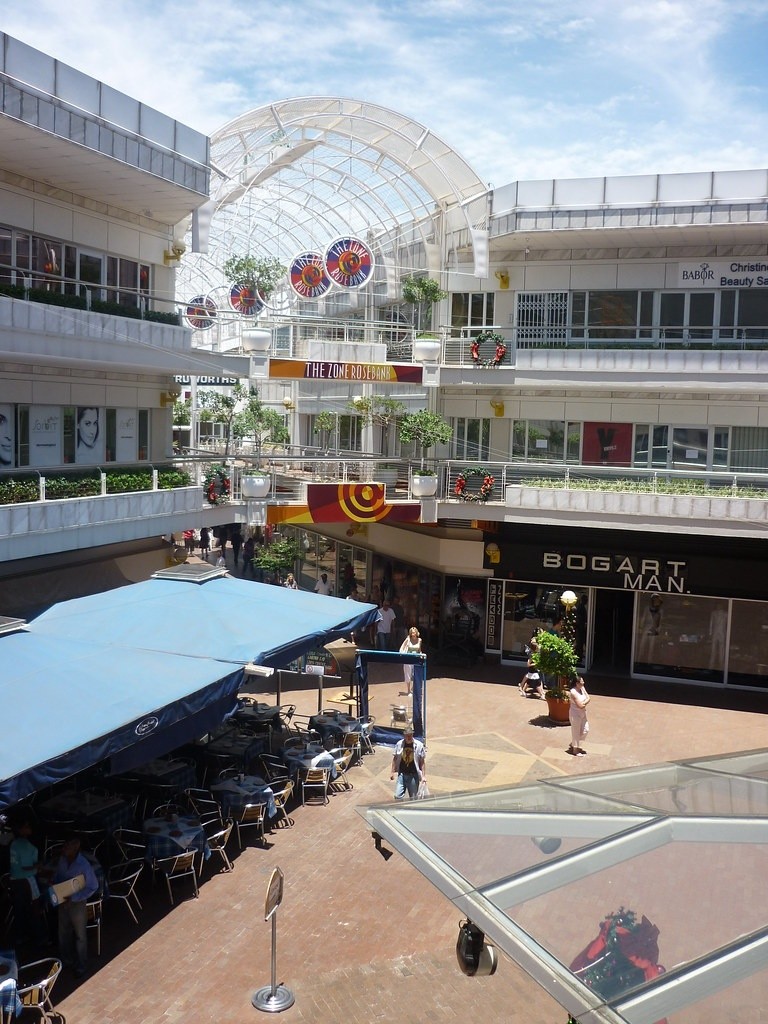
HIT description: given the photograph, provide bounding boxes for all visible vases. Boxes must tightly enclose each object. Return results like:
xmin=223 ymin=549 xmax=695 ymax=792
xmin=296 ymin=339 xmax=387 ymax=363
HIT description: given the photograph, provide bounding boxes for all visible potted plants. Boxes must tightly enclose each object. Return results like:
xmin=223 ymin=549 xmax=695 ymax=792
xmin=506 ymin=476 xmax=768 ymax=521
xmin=531 ymin=628 xmax=580 ymax=726
xmin=411 ymin=470 xmax=438 ymax=496
xmin=224 ymin=253 xmax=288 ymax=351
xmin=372 ymin=465 xmax=399 ymax=487
xmin=515 ymin=344 xmax=768 ymax=373
xmin=402 ymin=276 xmax=448 ymax=360
xmin=0 ymin=282 xmax=192 ymax=350
xmin=313 ymin=410 xmax=344 ymax=476
xmin=240 ymin=470 xmax=271 ymax=497
xmin=0 ymin=467 xmax=203 ymax=536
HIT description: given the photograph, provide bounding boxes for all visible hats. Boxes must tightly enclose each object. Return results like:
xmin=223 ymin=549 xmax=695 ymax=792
xmin=403 ymin=726 xmax=415 ymax=734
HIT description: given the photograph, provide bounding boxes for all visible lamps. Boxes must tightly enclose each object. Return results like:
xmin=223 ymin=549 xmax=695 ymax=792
xmin=174 ymin=547 xmax=188 ymax=563
xmin=490 ymin=395 xmax=505 ymax=418
xmin=282 ymin=396 xmax=296 ymax=411
xmin=346 ymin=522 xmax=369 ymax=538
xmin=371 ymin=830 xmax=393 ymax=862
xmin=160 ymin=382 xmax=182 ymax=407
xmin=531 ymin=836 xmax=562 ymax=854
xmin=164 ymin=239 xmax=187 ymax=265
xmin=456 ymin=916 xmax=499 ymax=977
xmin=354 ymin=396 xmax=370 ymax=412
xmin=494 ymin=265 xmax=509 ymax=289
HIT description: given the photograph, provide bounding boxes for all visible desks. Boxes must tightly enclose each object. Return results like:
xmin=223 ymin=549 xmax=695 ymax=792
xmin=238 ymin=703 xmax=283 ymax=742
xmin=127 ymin=759 xmax=196 ymax=806
xmin=281 ymin=743 xmax=339 ymax=789
xmin=312 ymin=713 xmax=362 ymax=748
xmin=142 ymin=814 xmax=211 ymax=873
xmin=202 ymin=731 xmax=264 ymax=773
xmin=211 ymin=775 xmax=278 ymax=827
xmin=37 ymin=791 xmax=131 ymax=854
xmin=327 ymin=691 xmax=375 ymax=717
xmin=505 ymin=593 xmax=528 ymax=619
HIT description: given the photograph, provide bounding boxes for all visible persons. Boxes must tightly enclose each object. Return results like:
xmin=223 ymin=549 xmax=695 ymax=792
xmin=77 ymin=406 xmax=99 ymax=448
xmin=399 ymin=627 xmax=422 ymax=694
xmin=39 ymin=835 xmax=100 ymax=975
xmin=344 ymin=583 xmax=407 ymax=651
xmin=388 ymin=726 xmax=428 ymax=801
xmin=568 ymin=676 xmax=591 ymax=757
xmin=708 ymin=602 xmax=729 ymax=669
xmin=9 ymin=818 xmax=42 ymax=945
xmin=313 ymin=573 xmax=334 ymax=596
xmin=282 ymin=573 xmax=299 ymax=589
xmin=0 ymin=405 xmax=12 ymax=469
xmin=183 ymin=526 xmax=264 ymax=572
xmin=518 ymin=618 xmax=562 ymax=700
xmin=637 ymin=593 xmax=663 ymax=662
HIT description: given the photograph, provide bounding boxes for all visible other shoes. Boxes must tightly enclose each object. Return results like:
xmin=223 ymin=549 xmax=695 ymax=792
xmin=407 ymin=691 xmax=413 ymax=697
xmin=572 ymin=752 xmax=583 ymax=756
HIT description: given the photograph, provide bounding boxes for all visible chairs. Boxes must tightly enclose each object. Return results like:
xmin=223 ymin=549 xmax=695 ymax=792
xmin=0 ymin=696 xmax=376 ymax=1024
xmin=390 ymin=705 xmax=413 ymax=731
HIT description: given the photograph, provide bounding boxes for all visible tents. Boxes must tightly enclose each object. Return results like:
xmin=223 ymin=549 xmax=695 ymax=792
xmin=0 ymin=578 xmax=385 ymax=807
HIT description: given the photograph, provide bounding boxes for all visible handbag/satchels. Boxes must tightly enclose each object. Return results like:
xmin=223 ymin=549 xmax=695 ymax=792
xmin=416 ymin=779 xmax=430 ymax=796
